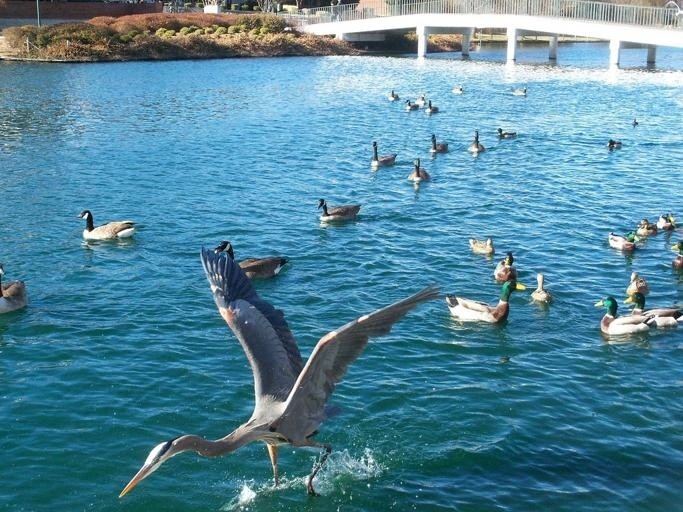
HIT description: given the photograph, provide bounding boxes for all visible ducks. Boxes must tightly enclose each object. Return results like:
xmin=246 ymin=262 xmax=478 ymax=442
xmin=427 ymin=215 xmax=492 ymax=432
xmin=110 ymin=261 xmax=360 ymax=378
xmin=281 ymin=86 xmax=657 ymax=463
xmin=446 ymin=278 xmax=527 ymax=323
xmin=593 ymin=212 xmax=683 ymax=335
xmin=469 ymin=238 xmax=495 ymax=254
xmin=531 ymin=273 xmax=552 ymax=304
xmin=494 ymin=252 xmax=518 ymax=282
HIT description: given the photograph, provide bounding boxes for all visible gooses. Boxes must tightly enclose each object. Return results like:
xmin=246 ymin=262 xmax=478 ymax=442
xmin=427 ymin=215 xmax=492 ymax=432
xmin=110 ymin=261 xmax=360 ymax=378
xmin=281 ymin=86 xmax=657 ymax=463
xmin=511 ymin=88 xmax=527 ymax=96
xmin=607 ymin=139 xmax=622 ymax=147
xmin=452 ymin=85 xmax=463 ymax=93
xmin=496 ymin=128 xmax=516 ymax=138
xmin=429 ymin=134 xmax=448 ymax=152
xmin=317 ymin=199 xmax=361 ymax=221
xmin=468 ymin=131 xmax=484 ymax=152
xmin=631 ymin=118 xmax=638 ymax=125
xmin=214 ymin=241 xmax=289 ymax=279
xmin=404 ymin=94 xmax=438 ymax=113
xmin=388 ymin=90 xmax=398 ymax=101
xmin=408 ymin=159 xmax=431 ymax=180
xmin=77 ymin=209 xmax=138 ymax=240
xmin=371 ymin=141 xmax=397 ymax=166
xmin=0 ymin=263 xmax=26 ymax=314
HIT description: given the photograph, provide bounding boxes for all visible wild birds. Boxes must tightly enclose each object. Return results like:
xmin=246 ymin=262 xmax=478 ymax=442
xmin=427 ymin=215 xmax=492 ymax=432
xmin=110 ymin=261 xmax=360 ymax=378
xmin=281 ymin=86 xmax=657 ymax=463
xmin=118 ymin=246 xmax=445 ymax=500
